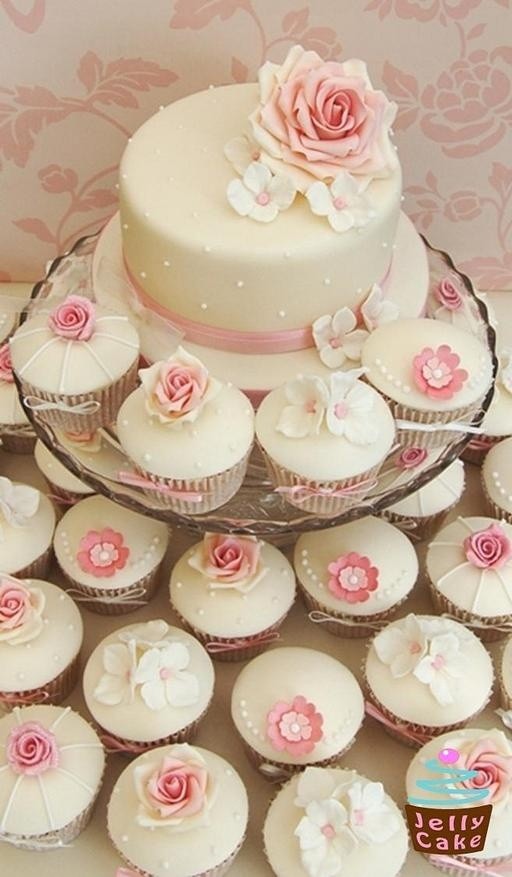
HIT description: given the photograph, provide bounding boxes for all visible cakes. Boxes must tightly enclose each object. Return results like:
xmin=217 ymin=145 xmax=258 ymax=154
xmin=0 ymin=44 xmax=512 ymax=877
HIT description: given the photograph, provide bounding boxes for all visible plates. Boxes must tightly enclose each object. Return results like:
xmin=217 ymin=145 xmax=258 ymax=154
xmin=8 ymin=212 xmax=501 ymax=539
